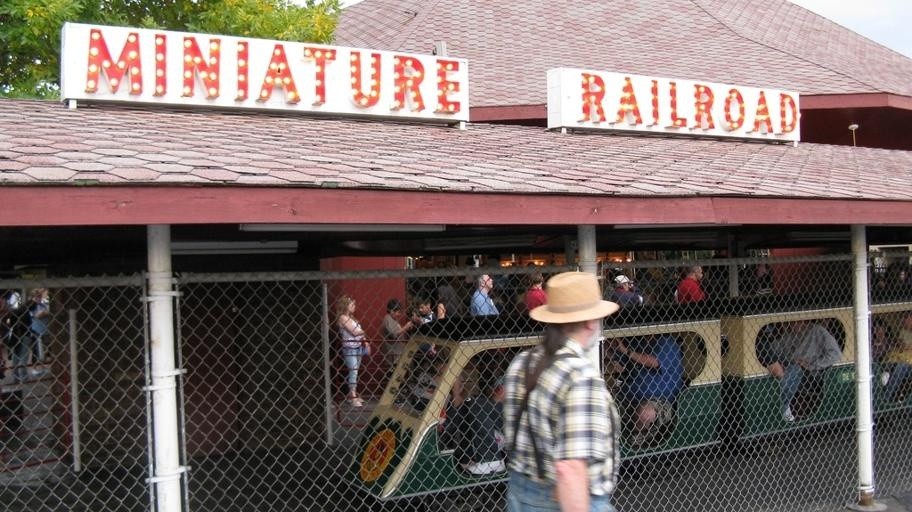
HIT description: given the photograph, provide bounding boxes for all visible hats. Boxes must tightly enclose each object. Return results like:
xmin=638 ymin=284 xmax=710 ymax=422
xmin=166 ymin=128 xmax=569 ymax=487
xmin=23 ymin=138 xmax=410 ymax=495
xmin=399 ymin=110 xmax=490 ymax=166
xmin=528 ymin=271 xmax=620 ymax=323
xmin=615 ymin=275 xmax=635 ymax=284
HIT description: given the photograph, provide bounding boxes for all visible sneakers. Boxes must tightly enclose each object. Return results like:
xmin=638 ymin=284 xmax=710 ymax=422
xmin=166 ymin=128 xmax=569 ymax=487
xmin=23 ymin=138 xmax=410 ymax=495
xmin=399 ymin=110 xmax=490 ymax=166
xmin=782 ymin=407 xmax=795 ymax=422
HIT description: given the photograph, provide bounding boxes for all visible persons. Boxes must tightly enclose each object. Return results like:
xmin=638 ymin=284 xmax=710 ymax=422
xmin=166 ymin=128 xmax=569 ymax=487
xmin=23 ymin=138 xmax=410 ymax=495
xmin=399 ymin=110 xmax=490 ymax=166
xmin=337 ymin=295 xmax=371 ymax=407
xmin=0 ymin=289 xmax=49 ymax=383
xmin=504 ymin=271 xmax=621 ymax=512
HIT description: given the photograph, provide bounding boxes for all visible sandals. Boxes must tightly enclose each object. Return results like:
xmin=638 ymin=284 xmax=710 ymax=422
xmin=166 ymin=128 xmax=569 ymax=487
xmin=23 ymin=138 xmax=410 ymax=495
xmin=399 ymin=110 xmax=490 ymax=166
xmin=347 ymin=396 xmax=366 ymax=407
